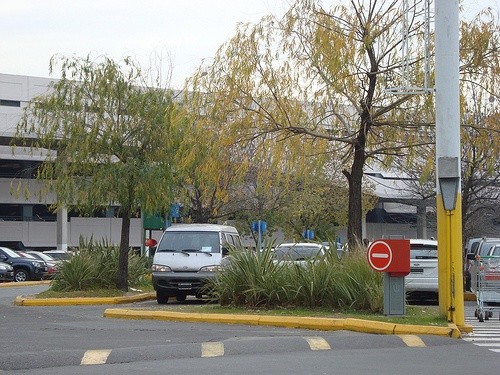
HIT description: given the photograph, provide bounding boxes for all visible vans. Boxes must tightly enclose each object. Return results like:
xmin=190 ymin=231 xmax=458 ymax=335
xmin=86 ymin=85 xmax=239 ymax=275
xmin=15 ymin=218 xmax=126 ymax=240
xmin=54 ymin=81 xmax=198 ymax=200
xmin=152 ymin=223 xmax=243 ymax=305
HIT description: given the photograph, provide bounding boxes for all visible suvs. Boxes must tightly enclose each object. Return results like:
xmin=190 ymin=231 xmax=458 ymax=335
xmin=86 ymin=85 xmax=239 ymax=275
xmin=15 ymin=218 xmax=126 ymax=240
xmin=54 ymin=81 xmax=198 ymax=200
xmin=0 ymin=246 xmax=47 ymax=282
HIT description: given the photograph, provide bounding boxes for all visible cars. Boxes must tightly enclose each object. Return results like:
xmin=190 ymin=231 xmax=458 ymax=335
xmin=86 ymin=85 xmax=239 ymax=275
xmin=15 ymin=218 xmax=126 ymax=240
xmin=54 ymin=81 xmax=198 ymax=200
xmin=15 ymin=251 xmax=35 ymax=259
xmin=272 ymin=243 xmax=331 ymax=269
xmin=465 ymin=236 xmax=500 ymax=291
xmin=0 ymin=262 xmax=14 ymax=283
xmin=323 ymin=242 xmax=349 ymax=259
xmin=43 ymin=250 xmax=71 ymax=267
xmin=24 ymin=251 xmax=61 ymax=280
xmin=406 ymin=236 xmax=439 ymax=302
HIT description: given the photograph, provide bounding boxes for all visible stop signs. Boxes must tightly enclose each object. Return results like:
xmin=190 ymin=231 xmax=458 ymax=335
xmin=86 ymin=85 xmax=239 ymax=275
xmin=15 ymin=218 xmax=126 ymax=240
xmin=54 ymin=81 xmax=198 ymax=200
xmin=367 ymin=240 xmax=410 ymax=272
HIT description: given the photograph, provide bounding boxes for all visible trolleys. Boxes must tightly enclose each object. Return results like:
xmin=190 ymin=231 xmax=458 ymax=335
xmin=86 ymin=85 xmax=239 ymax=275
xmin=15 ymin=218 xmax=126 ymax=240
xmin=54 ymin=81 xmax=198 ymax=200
xmin=470 ymin=254 xmax=500 ymax=322
